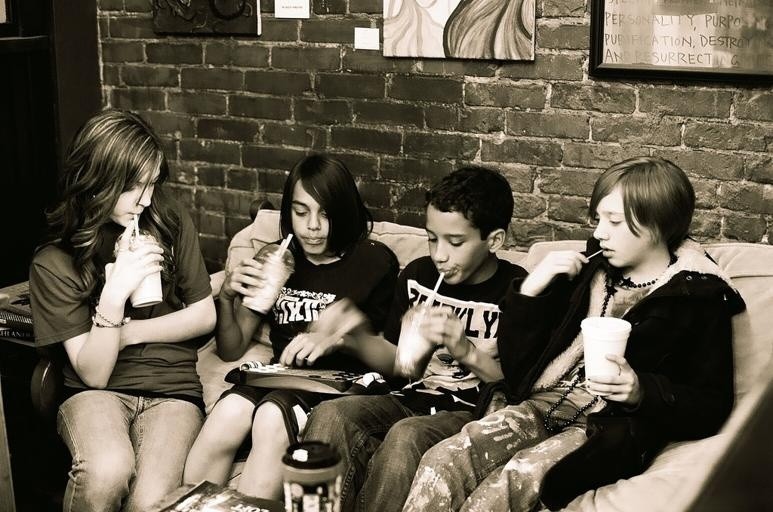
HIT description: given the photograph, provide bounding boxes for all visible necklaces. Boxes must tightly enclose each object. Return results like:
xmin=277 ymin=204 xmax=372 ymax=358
xmin=544 ymin=269 xmax=659 ymax=432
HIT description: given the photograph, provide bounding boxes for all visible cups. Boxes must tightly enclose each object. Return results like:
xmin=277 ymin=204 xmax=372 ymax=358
xmin=241 ymin=242 xmax=294 ymax=314
xmin=116 ymin=230 xmax=163 ymax=309
xmin=578 ymin=317 xmax=631 ymax=394
xmin=279 ymin=441 xmax=344 ymax=511
xmin=393 ymin=304 xmax=441 ymax=381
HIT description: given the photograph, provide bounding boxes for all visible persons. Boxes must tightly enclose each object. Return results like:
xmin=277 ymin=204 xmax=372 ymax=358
xmin=401 ymin=155 xmax=748 ymax=512
xmin=27 ymin=107 xmax=218 ymax=512
xmin=183 ymin=153 xmax=405 ymax=501
xmin=302 ymin=165 xmax=530 ymax=512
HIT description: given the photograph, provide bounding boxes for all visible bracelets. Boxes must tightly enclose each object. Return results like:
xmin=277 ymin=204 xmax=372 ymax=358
xmin=92 ymin=306 xmax=130 ymax=329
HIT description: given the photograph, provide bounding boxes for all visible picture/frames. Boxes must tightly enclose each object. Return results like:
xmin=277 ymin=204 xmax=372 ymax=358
xmin=588 ymin=0 xmax=773 ymax=89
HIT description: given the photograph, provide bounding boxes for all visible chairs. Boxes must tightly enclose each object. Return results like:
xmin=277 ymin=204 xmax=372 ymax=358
xmin=30 ymin=354 xmax=75 ymax=511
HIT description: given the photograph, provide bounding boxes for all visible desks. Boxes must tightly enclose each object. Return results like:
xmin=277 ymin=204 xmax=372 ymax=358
xmin=0 ymin=335 xmax=53 ymax=512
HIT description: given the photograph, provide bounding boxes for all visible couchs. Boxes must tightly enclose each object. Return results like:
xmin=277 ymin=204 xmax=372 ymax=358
xmin=183 ymin=199 xmax=773 ymax=512
